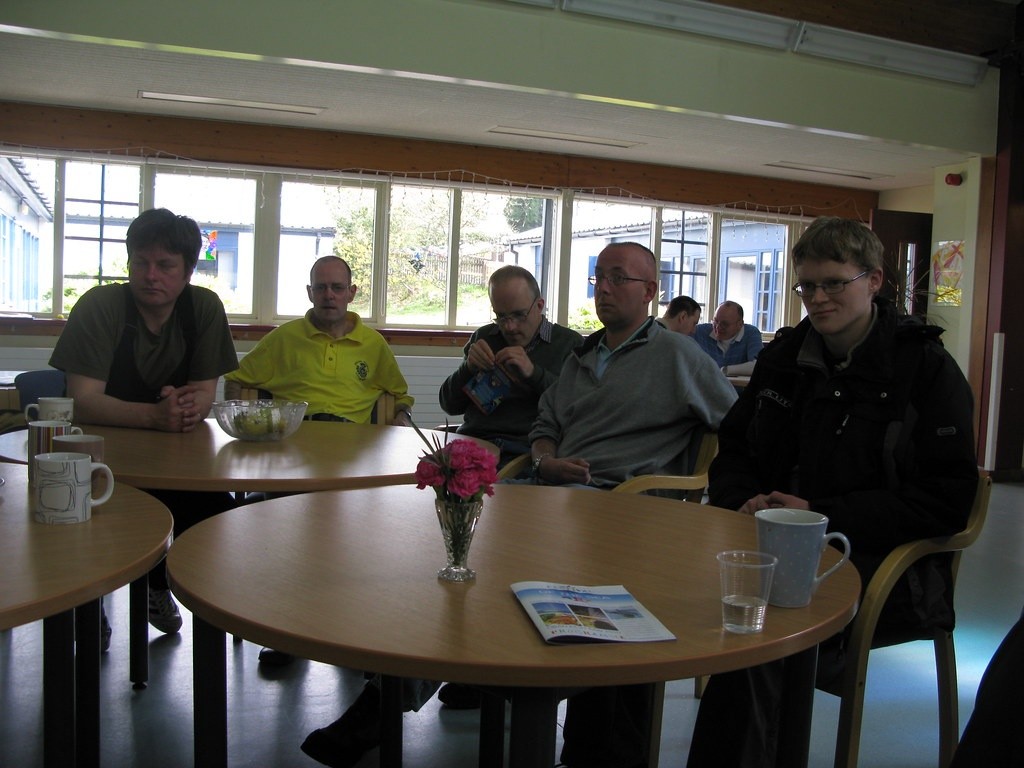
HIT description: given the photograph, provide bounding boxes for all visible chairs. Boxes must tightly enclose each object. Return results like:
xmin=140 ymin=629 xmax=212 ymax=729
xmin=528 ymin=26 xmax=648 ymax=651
xmin=496 ymin=426 xmax=720 ymax=504
xmin=814 ymin=465 xmax=993 ymax=768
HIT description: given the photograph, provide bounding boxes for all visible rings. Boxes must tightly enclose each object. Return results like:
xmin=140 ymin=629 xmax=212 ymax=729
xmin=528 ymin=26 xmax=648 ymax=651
xmin=197 ymin=413 xmax=201 ymax=420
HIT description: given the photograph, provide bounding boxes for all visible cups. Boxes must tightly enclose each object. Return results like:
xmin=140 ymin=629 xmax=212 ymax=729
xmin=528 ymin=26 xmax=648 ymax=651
xmin=28 ymin=421 xmax=84 ymax=481
xmin=51 ymin=435 xmax=105 ymax=494
xmin=25 ymin=398 xmax=74 ymax=425
xmin=716 ymin=550 xmax=776 ymax=635
xmin=34 ymin=453 xmax=114 ymax=525
xmin=754 ymin=508 xmax=849 ymax=609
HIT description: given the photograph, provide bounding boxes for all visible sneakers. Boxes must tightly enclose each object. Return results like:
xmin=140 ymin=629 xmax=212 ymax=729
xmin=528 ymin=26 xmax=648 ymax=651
xmin=99 ymin=615 xmax=113 ymax=650
xmin=147 ymin=586 xmax=185 ymax=634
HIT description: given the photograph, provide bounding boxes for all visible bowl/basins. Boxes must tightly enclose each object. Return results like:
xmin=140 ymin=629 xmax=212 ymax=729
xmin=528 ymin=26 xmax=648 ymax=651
xmin=209 ymin=398 xmax=309 ymax=442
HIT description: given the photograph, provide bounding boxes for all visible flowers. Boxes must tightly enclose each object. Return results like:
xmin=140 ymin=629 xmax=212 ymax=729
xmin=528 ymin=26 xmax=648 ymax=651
xmin=399 ymin=408 xmax=497 ymax=569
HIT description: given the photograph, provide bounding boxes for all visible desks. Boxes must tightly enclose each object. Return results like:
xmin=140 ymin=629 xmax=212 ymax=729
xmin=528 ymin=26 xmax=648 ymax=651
xmin=0 ymin=414 xmax=500 ymax=688
xmin=0 ymin=461 xmax=175 ymax=767
xmin=162 ymin=482 xmax=860 ymax=768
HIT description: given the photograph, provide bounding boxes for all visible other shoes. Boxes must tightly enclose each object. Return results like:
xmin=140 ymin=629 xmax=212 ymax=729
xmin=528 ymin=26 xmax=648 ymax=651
xmin=438 ymin=681 xmax=483 ymax=709
xmin=300 ymin=682 xmax=381 ymax=768
xmin=257 ymin=646 xmax=308 ymax=668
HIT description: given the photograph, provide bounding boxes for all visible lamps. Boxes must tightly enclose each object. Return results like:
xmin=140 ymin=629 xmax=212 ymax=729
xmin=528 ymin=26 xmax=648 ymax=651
xmin=505 ymin=0 xmax=991 ymax=88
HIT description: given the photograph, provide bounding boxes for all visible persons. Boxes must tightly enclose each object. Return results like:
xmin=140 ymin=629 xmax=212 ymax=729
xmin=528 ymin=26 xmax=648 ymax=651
xmin=552 ymin=216 xmax=977 ymax=767
xmin=432 ymin=265 xmax=585 ymax=702
xmin=299 ymin=242 xmax=740 ymax=768
xmin=47 ymin=210 xmax=239 ymax=654
xmin=654 ymin=296 xmax=766 ymax=495
xmin=226 ymin=256 xmax=415 ymax=664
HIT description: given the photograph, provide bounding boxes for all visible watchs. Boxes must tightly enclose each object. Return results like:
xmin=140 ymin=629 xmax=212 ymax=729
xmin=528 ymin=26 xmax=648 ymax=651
xmin=721 ymin=366 xmax=728 ymax=376
xmin=394 ymin=407 xmax=413 ymax=421
xmin=531 ymin=453 xmax=554 ymax=478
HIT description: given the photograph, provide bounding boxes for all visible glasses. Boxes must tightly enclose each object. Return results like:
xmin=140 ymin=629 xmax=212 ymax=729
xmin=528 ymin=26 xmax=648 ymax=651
xmin=587 ymin=272 xmax=650 ymax=287
xmin=493 ymin=294 xmax=539 ymax=327
xmin=794 ymin=266 xmax=877 ymax=297
xmin=712 ymin=317 xmax=742 ymax=329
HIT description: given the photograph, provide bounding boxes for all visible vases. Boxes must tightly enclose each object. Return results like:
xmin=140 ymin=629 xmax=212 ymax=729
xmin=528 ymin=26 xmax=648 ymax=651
xmin=433 ymin=497 xmax=486 ymax=583
